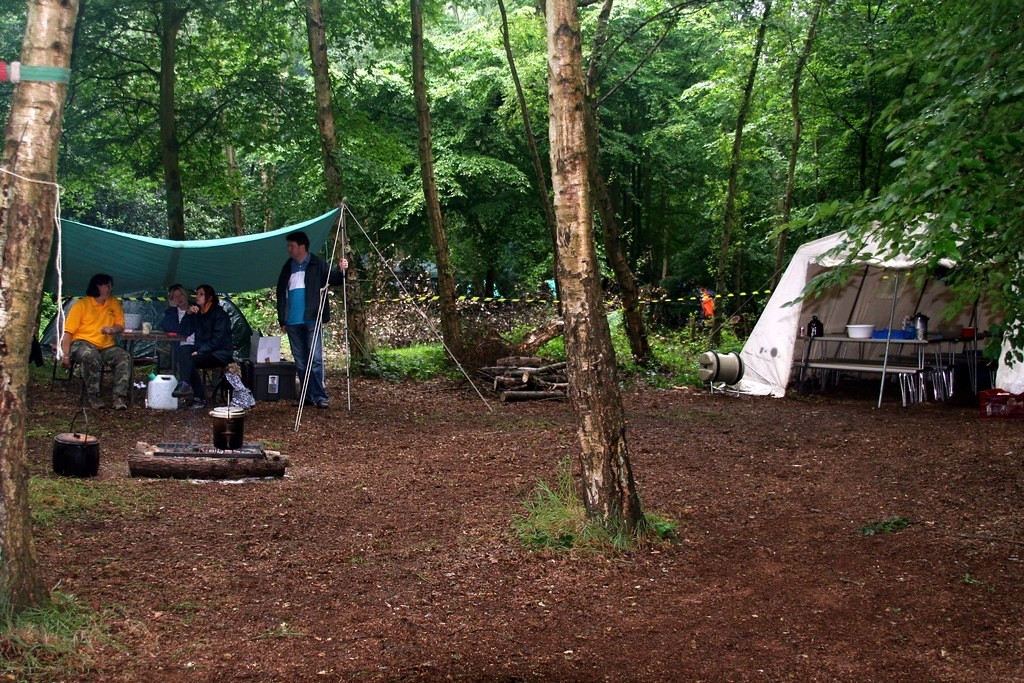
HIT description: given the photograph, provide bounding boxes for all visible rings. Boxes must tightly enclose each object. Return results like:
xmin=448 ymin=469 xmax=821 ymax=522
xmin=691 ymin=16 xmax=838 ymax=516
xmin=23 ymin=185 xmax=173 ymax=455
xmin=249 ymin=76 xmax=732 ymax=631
xmin=107 ymin=330 xmax=109 ymax=332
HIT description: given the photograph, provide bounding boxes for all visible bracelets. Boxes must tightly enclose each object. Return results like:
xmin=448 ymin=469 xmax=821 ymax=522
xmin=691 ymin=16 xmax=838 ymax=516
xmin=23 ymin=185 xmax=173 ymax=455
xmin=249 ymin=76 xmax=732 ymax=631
xmin=110 ymin=327 xmax=115 ymax=333
xmin=63 ymin=355 xmax=70 ymax=359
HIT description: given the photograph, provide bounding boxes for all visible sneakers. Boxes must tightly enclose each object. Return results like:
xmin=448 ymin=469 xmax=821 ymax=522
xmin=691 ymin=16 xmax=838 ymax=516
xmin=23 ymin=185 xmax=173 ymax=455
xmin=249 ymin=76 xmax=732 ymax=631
xmin=172 ymin=383 xmax=194 ymax=396
xmin=89 ymin=398 xmax=105 ymax=409
xmin=113 ymin=398 xmax=127 ymax=410
xmin=184 ymin=397 xmax=207 ymax=410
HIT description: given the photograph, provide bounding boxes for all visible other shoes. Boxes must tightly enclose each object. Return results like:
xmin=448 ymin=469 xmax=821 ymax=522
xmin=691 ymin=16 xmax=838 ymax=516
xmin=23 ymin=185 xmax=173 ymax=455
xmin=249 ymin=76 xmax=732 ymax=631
xmin=292 ymin=399 xmax=314 ymax=407
xmin=317 ymin=400 xmax=329 ymax=409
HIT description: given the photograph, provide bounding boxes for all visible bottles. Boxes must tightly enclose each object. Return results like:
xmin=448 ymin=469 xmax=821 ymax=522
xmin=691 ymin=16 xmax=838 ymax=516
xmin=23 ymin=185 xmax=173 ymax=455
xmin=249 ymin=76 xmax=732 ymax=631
xmin=913 ymin=312 xmax=929 ymax=339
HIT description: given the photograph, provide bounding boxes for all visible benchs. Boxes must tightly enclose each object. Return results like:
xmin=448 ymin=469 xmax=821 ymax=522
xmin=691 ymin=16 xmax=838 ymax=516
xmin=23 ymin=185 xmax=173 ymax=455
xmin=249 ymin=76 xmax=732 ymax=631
xmin=794 ymin=350 xmax=997 ymax=407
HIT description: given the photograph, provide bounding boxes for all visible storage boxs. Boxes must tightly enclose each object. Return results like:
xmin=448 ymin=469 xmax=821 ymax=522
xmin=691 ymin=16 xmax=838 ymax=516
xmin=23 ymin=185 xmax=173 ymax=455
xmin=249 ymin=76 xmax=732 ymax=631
xmin=254 ymin=360 xmax=296 ymax=401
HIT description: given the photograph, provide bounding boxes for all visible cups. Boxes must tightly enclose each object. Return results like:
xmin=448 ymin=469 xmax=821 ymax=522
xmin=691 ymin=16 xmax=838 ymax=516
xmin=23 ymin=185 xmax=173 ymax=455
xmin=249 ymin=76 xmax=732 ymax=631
xmin=142 ymin=322 xmax=152 ymax=334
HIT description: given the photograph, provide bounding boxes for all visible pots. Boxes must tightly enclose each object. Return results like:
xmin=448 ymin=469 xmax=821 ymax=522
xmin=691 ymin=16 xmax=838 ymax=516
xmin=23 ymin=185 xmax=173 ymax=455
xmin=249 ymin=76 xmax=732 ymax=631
xmin=53 ymin=407 xmax=99 ymax=478
xmin=210 ymin=388 xmax=246 ymax=450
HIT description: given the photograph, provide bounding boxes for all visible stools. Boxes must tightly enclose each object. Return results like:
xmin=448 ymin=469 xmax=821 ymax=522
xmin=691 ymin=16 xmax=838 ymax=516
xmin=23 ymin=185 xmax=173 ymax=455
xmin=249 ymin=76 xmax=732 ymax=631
xmin=200 ymin=362 xmax=242 ymax=404
xmin=52 ymin=346 xmax=117 ymax=407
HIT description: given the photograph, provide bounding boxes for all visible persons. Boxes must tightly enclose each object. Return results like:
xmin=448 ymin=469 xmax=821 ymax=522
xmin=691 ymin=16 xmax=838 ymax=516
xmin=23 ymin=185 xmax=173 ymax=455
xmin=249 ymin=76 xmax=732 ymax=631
xmin=60 ymin=273 xmax=132 ymax=410
xmin=171 ymin=283 xmax=233 ymax=408
xmin=162 ymin=283 xmax=197 ymax=361
xmin=276 ymin=231 xmax=349 ymax=408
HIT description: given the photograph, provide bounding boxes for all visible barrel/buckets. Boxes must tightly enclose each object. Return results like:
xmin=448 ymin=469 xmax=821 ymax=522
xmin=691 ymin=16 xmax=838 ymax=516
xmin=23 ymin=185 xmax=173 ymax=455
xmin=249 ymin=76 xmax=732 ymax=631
xmin=147 ymin=373 xmax=179 ymax=411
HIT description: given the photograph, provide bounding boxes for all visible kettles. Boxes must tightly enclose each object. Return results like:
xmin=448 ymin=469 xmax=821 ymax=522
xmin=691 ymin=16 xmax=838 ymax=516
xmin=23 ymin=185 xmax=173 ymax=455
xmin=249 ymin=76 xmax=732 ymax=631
xmin=807 ymin=316 xmax=823 ymax=338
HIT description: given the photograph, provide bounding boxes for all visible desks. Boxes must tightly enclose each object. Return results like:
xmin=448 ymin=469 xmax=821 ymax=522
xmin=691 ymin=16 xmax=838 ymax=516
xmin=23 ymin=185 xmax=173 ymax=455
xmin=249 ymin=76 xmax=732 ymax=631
xmin=118 ymin=330 xmax=186 ymax=406
xmin=796 ymin=333 xmax=992 ymax=403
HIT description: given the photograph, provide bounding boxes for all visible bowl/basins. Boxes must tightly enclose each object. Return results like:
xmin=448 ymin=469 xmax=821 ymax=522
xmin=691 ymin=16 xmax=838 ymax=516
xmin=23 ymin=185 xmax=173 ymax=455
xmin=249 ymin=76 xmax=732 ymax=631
xmin=937 ymin=324 xmax=963 ymax=338
xmin=962 ymin=327 xmax=977 ymax=337
xmin=121 ymin=314 xmax=141 ymax=330
xmin=846 ymin=324 xmax=877 ymax=340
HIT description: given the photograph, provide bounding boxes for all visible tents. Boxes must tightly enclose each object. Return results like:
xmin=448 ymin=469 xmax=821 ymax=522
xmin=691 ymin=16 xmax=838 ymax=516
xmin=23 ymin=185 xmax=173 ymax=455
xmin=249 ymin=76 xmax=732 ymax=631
xmin=721 ymin=210 xmax=1024 ymax=416
xmin=38 ymin=288 xmax=254 ymax=362
